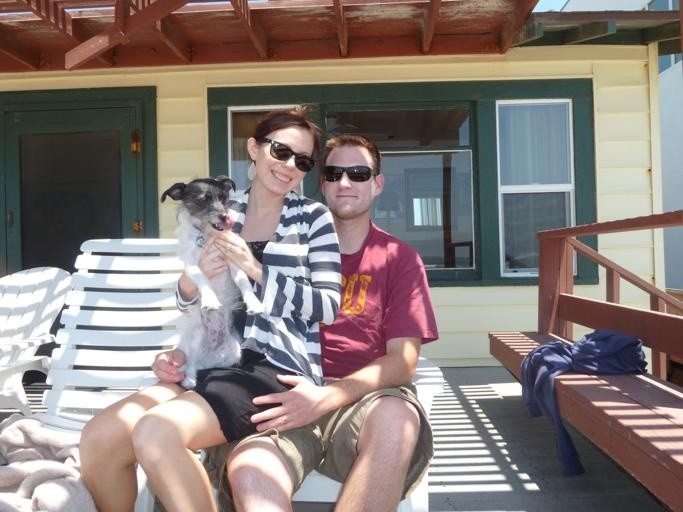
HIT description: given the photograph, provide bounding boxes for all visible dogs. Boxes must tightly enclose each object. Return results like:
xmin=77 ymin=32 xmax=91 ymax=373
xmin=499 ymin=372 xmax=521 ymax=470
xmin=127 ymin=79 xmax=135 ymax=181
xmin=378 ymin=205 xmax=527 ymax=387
xmin=160 ymin=176 xmax=264 ymax=388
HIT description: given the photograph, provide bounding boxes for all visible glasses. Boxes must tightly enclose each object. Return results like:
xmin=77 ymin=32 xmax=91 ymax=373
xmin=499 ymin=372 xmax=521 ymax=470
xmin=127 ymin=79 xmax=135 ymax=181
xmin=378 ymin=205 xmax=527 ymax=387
xmin=257 ymin=137 xmax=317 ymax=173
xmin=322 ymin=165 xmax=373 ymax=182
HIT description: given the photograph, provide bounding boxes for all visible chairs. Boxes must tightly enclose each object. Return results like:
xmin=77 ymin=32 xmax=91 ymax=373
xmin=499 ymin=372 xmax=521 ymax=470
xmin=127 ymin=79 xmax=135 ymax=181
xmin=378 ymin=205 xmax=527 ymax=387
xmin=1 ymin=238 xmax=180 ymax=511
xmin=292 ymin=357 xmax=444 ymax=512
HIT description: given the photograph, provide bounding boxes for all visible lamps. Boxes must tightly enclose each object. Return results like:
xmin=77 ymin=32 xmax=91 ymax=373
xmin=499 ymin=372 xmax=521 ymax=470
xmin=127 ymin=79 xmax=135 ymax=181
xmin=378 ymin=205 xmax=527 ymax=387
xmin=376 ymin=191 xmax=400 ymax=232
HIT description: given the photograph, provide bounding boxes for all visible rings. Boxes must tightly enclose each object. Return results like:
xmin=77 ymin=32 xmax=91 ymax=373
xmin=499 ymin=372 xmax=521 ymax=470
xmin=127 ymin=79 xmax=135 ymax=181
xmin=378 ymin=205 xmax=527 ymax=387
xmin=283 ymin=415 xmax=287 ymax=424
xmin=227 ymin=243 xmax=232 ymax=250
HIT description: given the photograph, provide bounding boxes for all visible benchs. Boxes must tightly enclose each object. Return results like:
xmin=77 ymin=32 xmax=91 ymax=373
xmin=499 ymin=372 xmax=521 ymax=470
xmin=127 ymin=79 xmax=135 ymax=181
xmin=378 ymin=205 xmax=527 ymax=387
xmin=488 ymin=329 xmax=683 ymax=512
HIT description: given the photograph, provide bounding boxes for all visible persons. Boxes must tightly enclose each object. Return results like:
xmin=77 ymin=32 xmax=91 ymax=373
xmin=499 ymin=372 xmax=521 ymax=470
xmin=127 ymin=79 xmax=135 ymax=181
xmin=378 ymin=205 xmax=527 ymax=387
xmin=79 ymin=106 xmax=342 ymax=512
xmin=226 ymin=135 xmax=436 ymax=512
